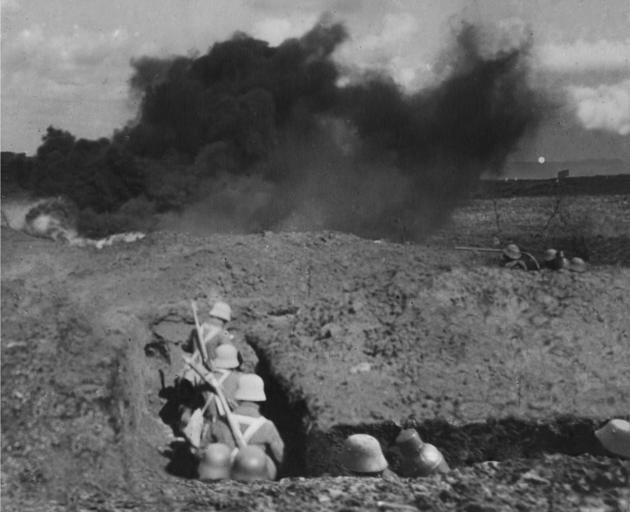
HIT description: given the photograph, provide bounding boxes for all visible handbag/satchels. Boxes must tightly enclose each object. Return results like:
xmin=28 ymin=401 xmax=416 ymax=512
xmin=182 ymin=406 xmax=204 ymax=454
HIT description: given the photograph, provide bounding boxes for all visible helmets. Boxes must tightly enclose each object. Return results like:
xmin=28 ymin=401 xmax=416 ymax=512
xmin=542 ymin=247 xmax=557 ymax=262
xmin=196 ymin=442 xmax=233 ymax=482
xmin=231 ymin=444 xmax=277 ymax=482
xmin=214 ymin=343 xmax=240 ymax=370
xmin=208 ymin=301 xmax=233 ymax=324
xmin=233 ymin=373 xmax=267 ymax=403
xmin=502 ymin=244 xmax=522 ymax=260
xmin=567 ymin=256 xmax=585 ymax=272
xmin=341 ymin=432 xmax=391 ymax=475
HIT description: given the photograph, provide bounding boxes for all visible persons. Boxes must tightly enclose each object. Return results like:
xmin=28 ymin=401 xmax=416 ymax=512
xmin=182 ymin=292 xmax=297 ymax=485
xmin=339 ymin=431 xmax=388 ymax=478
xmin=498 ymin=243 xmax=588 ymax=273
